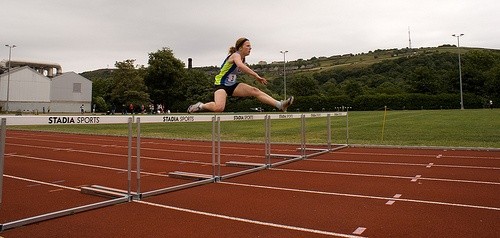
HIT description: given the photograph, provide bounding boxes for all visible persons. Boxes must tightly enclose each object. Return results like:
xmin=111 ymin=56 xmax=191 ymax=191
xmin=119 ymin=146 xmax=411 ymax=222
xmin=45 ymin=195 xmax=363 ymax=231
xmin=80 ymin=103 xmax=85 ymax=114
xmin=121 ymin=103 xmax=127 ymax=115
xmin=141 ymin=103 xmax=165 ymax=114
xmin=110 ymin=103 xmax=116 ymax=115
xmin=186 ymin=39 xmax=294 ymax=114
xmin=130 ymin=102 xmax=135 ymax=114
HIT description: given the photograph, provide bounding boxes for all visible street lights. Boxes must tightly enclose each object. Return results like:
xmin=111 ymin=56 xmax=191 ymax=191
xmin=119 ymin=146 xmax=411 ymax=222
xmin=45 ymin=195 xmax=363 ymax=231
xmin=451 ymin=33 xmax=465 ymax=111
xmin=5 ymin=44 xmax=16 ymax=114
xmin=279 ymin=50 xmax=288 ymax=113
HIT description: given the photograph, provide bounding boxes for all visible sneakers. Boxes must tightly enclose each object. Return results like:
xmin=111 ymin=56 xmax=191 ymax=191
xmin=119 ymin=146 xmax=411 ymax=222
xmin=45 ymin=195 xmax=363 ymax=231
xmin=187 ymin=101 xmax=203 ymax=113
xmin=281 ymin=96 xmax=294 ymax=111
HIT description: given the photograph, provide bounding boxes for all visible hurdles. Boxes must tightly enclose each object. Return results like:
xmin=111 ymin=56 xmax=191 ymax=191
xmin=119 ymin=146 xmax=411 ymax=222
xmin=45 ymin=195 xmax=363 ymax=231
xmin=269 ymin=113 xmax=331 ymax=159
xmin=301 ymin=112 xmax=349 ymax=152
xmin=229 ymin=114 xmax=304 ymax=168
xmin=0 ymin=115 xmax=133 ymax=232
xmin=91 ymin=115 xmax=218 ymax=200
xmin=174 ymin=114 xmax=269 ymax=182
